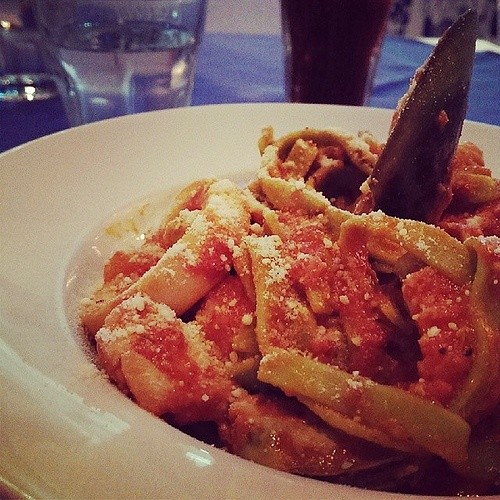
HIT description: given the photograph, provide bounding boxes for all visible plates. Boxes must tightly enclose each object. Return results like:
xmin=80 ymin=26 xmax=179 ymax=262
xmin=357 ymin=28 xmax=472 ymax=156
xmin=0 ymin=102 xmax=500 ymax=500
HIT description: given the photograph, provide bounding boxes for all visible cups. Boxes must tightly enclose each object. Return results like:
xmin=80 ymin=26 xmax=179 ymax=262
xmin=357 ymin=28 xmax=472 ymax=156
xmin=31 ymin=0 xmax=207 ymax=127
xmin=279 ymin=0 xmax=393 ymax=106
